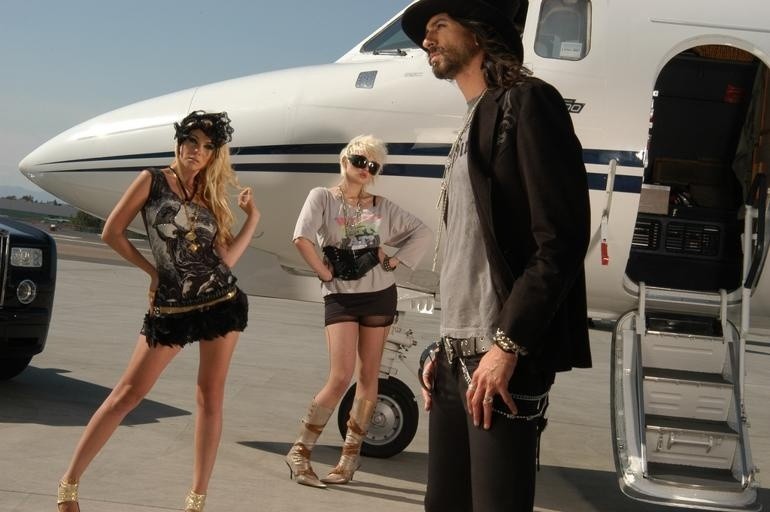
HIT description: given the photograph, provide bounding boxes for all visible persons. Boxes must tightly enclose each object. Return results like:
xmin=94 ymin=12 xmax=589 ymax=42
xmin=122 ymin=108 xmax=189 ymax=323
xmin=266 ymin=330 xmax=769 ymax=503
xmin=283 ymin=132 xmax=435 ymax=488
xmin=55 ymin=109 xmax=260 ymax=512
xmin=398 ymin=1 xmax=594 ymax=512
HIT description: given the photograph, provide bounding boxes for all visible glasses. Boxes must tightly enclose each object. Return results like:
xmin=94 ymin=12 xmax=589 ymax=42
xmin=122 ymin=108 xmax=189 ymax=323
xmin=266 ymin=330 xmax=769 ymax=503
xmin=348 ymin=155 xmax=380 ymax=176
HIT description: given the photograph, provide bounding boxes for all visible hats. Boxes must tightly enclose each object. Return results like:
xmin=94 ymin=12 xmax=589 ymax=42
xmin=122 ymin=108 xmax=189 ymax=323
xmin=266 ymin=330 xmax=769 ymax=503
xmin=400 ymin=1 xmax=529 ymax=52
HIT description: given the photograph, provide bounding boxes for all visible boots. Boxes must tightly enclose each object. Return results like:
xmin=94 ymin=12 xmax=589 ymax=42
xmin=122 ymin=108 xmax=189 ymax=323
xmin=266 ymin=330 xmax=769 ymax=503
xmin=284 ymin=395 xmax=334 ymax=488
xmin=320 ymin=396 xmax=375 ymax=484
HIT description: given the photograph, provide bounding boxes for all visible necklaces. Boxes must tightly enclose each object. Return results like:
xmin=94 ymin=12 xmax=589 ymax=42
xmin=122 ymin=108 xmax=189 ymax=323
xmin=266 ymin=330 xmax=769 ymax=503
xmin=428 ymin=85 xmax=486 ymax=271
xmin=169 ymin=166 xmax=201 ymax=242
xmin=169 ymin=164 xmax=199 ymax=207
xmin=335 ymin=183 xmax=363 ymax=240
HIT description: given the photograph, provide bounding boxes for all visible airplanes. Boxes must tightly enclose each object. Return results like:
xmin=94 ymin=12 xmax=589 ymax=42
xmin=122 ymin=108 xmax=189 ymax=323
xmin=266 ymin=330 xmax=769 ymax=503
xmin=19 ymin=2 xmax=770 ymax=457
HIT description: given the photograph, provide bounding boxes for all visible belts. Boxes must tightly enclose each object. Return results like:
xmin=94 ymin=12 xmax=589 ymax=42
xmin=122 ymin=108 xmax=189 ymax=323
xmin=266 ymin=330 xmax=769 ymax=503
xmin=440 ymin=334 xmax=495 ymax=364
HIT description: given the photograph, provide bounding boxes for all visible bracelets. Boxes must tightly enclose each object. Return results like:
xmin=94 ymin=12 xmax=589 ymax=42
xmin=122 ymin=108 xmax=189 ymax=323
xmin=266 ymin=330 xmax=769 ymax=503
xmin=381 ymin=255 xmax=397 ymax=273
xmin=491 ymin=328 xmax=528 ymax=358
xmin=317 ymin=273 xmax=335 ymax=284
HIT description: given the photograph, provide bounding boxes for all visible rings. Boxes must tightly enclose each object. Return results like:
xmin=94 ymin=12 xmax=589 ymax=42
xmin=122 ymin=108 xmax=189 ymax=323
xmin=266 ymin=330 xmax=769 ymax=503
xmin=482 ymin=394 xmax=494 ymax=403
xmin=467 ymin=380 xmax=475 ymax=391
